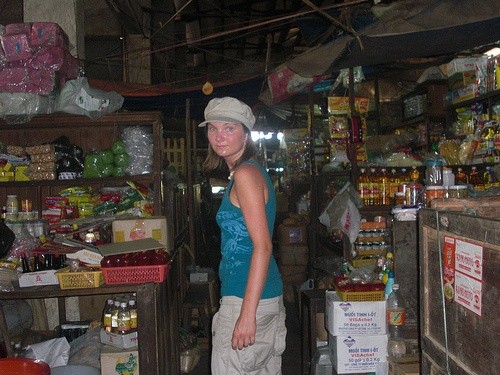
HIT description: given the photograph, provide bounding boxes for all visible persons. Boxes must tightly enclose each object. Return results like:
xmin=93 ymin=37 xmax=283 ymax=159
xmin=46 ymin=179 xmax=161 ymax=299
xmin=198 ymin=97 xmax=287 ymax=375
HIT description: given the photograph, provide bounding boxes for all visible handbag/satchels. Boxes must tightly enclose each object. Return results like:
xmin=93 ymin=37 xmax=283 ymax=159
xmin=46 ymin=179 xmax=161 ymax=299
xmin=0 ymin=218 xmax=15 ymax=259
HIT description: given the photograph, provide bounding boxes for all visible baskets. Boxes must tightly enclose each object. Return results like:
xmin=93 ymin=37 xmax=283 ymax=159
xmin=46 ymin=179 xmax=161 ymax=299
xmin=100 ymin=261 xmax=169 ymax=284
xmin=54 ymin=266 xmax=105 ymax=289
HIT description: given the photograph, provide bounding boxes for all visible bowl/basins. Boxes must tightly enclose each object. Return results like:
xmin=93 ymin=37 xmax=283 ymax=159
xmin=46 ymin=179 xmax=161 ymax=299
xmin=443 ymin=283 xmax=455 ymax=301
xmin=50 ymin=364 xmax=101 ymax=375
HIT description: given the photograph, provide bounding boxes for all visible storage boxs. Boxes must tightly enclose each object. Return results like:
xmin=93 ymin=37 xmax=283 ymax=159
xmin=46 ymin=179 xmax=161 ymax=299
xmin=404 ymin=94 xmax=425 ymax=119
xmin=447 ymin=58 xmax=475 ymax=77
xmin=190 ymin=270 xmax=219 ymax=326
xmin=99 ymin=329 xmax=139 ymax=375
xmin=111 ymin=215 xmax=171 ymax=251
xmin=18 ymin=269 xmax=59 ymax=288
xmin=447 ymin=71 xmax=475 ymax=93
xmin=279 ymin=224 xmax=308 ymax=284
xmin=0 ymin=163 xmax=32 ymax=182
xmin=325 ymin=290 xmax=422 ymax=375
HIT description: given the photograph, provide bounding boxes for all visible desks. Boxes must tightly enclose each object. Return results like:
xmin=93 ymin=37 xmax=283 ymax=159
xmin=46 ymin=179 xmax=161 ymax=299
xmin=417 ymin=196 xmax=500 ymax=375
xmin=0 ymin=281 xmax=179 ymax=375
xmin=301 ymin=288 xmax=327 ymax=375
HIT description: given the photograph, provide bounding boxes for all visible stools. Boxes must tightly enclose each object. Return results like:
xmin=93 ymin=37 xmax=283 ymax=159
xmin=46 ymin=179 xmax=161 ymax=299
xmin=182 ymin=284 xmax=213 ymax=336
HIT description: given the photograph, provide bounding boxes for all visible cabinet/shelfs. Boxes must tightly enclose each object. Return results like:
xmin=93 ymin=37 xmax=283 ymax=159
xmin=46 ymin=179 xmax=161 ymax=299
xmin=0 ymin=112 xmax=164 ymax=236
xmin=287 ymin=43 xmax=500 ymax=283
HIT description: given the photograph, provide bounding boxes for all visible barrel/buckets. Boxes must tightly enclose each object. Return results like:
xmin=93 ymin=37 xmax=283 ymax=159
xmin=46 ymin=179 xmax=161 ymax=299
xmin=0 ymin=357 xmax=50 ymax=375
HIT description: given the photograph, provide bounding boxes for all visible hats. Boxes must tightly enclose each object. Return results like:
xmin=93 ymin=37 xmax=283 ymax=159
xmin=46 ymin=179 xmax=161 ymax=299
xmin=197 ymin=96 xmax=256 ymax=131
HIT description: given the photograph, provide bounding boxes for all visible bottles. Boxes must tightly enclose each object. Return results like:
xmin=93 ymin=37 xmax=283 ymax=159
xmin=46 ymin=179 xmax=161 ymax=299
xmin=19 ymin=252 xmax=68 ymax=274
xmin=9 ymin=341 xmax=27 ymax=358
xmin=376 ymin=250 xmax=395 ymax=301
xmin=1 ymin=195 xmax=44 ymax=238
xmin=357 ymin=158 xmax=494 ymax=206
xmin=57 ymin=200 xmax=103 ymax=246
xmin=103 ymin=295 xmax=140 ymax=335
xmin=386 ymin=284 xmax=407 ymax=359
